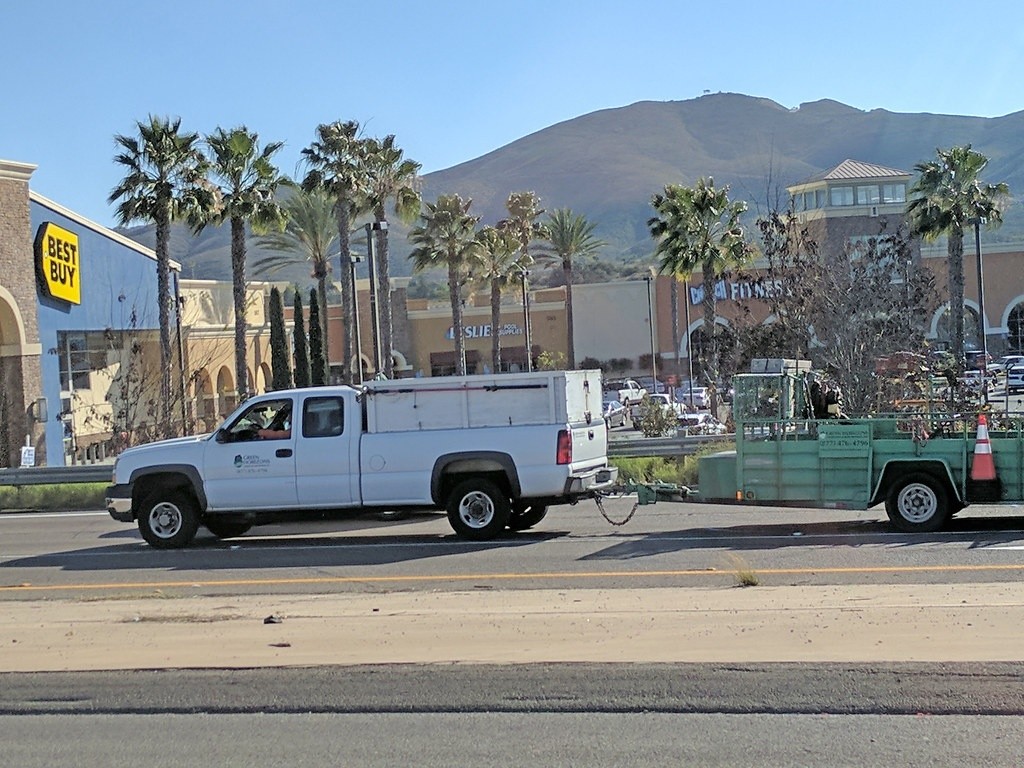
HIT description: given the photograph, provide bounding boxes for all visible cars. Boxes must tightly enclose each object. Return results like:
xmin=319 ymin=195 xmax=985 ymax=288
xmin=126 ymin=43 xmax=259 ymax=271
xmin=601 ymin=400 xmax=630 ymax=429
xmin=988 ymin=356 xmax=1024 ymax=375
xmin=684 ymin=387 xmax=714 ymax=409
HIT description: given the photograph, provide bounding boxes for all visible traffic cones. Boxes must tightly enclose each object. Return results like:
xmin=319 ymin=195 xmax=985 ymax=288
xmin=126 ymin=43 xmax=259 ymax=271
xmin=968 ymin=416 xmax=1000 ymax=483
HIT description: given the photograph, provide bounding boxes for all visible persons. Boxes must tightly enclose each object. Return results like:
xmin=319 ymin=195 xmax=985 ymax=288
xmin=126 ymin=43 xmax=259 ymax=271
xmin=757 ymin=384 xmax=783 ymax=418
xmin=258 ymin=408 xmax=291 ymax=438
xmin=802 ymin=382 xmax=852 ymax=425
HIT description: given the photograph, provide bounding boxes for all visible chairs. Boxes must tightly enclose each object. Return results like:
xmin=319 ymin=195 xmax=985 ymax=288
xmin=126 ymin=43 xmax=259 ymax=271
xmin=304 ymin=410 xmax=343 ymax=436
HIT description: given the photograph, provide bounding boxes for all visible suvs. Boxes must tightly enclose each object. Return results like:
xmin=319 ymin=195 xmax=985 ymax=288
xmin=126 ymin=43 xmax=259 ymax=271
xmin=601 ymin=378 xmax=648 ymax=405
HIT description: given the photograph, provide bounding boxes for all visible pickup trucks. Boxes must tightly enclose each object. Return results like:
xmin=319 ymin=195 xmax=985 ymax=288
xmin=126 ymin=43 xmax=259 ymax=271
xmin=628 ymin=394 xmax=686 ymax=426
xmin=105 ymin=386 xmax=618 ymax=549
